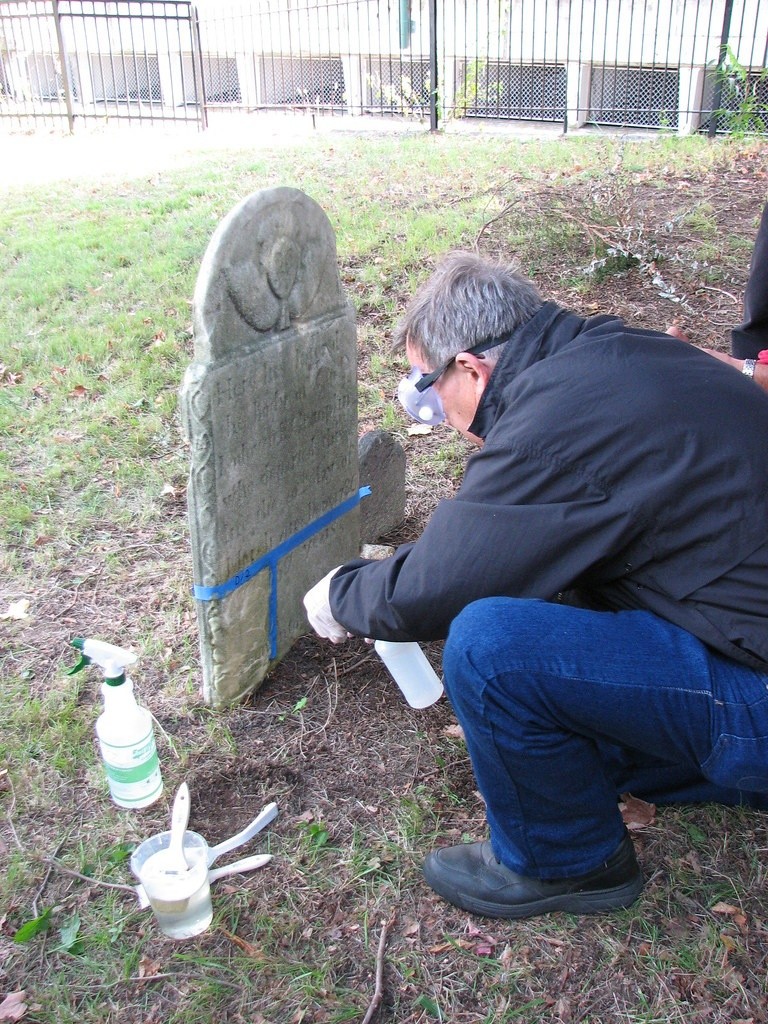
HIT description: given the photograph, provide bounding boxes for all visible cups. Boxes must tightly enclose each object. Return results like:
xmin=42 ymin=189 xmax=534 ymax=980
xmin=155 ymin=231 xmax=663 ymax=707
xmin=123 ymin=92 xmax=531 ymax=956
xmin=131 ymin=830 xmax=214 ymax=940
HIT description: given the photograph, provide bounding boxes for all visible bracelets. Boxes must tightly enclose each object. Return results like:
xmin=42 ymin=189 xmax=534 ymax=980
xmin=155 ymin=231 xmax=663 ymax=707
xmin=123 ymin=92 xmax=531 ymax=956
xmin=741 ymin=359 xmax=756 ymax=379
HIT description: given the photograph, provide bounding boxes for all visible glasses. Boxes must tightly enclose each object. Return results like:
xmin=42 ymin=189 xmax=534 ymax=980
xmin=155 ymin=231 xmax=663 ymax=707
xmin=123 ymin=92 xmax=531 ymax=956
xmin=397 ymin=368 xmax=445 ymax=426
xmin=415 ymin=353 xmax=485 ymax=416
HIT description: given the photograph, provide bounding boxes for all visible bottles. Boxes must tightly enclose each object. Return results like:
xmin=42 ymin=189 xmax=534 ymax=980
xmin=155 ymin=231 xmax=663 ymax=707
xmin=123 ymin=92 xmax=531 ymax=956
xmin=375 ymin=640 xmax=445 ymax=708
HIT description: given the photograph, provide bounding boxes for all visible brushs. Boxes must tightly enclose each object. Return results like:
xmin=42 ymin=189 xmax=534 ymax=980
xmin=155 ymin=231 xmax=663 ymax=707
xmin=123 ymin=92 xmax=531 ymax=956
xmin=135 ymin=852 xmax=273 ymax=911
xmin=152 ymin=777 xmax=191 ymax=912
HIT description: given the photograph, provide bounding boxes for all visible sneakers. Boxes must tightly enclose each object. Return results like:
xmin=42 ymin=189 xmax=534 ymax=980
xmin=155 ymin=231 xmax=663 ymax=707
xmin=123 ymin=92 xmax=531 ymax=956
xmin=423 ymin=832 xmax=641 ymax=919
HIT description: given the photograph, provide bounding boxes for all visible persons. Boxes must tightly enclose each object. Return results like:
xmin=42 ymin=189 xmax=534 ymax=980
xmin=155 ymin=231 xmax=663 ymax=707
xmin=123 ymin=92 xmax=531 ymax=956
xmin=664 ymin=200 xmax=768 ymax=394
xmin=304 ymin=251 xmax=768 ymax=919
